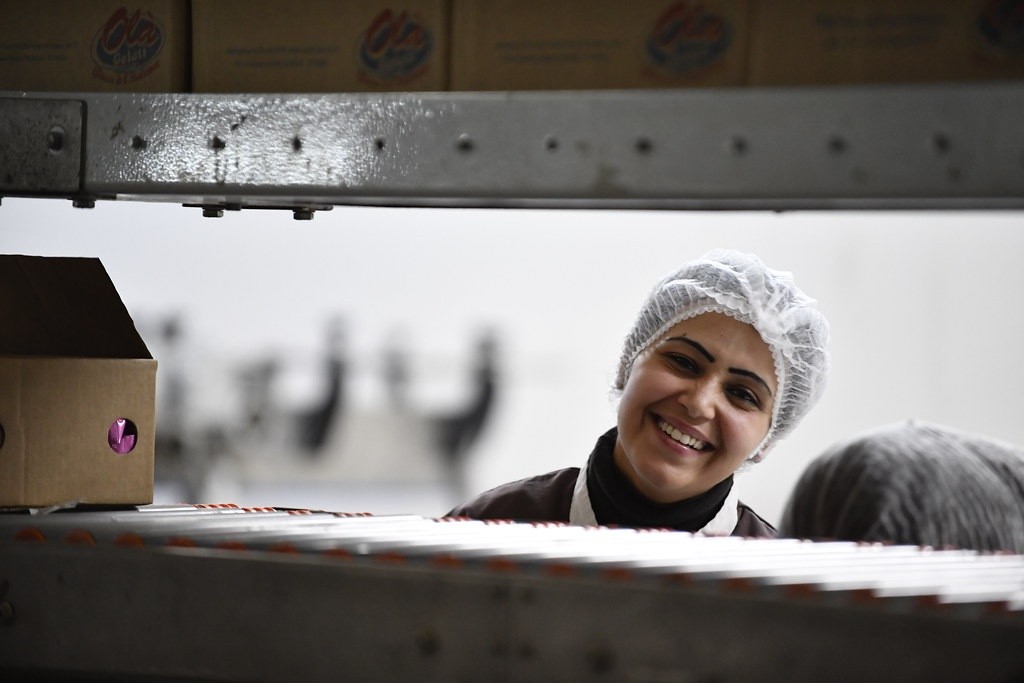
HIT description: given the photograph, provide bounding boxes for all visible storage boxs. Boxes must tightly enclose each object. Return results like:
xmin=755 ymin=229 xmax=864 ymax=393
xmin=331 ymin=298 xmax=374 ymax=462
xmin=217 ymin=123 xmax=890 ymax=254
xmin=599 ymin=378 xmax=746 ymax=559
xmin=0 ymin=0 xmax=189 ymax=92
xmin=748 ymin=0 xmax=1024 ymax=84
xmin=191 ymin=0 xmax=449 ymax=93
xmin=0 ymin=254 xmax=158 ymax=510
xmin=449 ymin=0 xmax=749 ymax=91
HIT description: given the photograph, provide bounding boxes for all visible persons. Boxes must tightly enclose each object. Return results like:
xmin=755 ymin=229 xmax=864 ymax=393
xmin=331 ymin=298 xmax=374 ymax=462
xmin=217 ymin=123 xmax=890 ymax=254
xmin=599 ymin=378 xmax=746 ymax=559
xmin=776 ymin=424 xmax=1024 ymax=554
xmin=442 ymin=247 xmax=827 ymax=538
